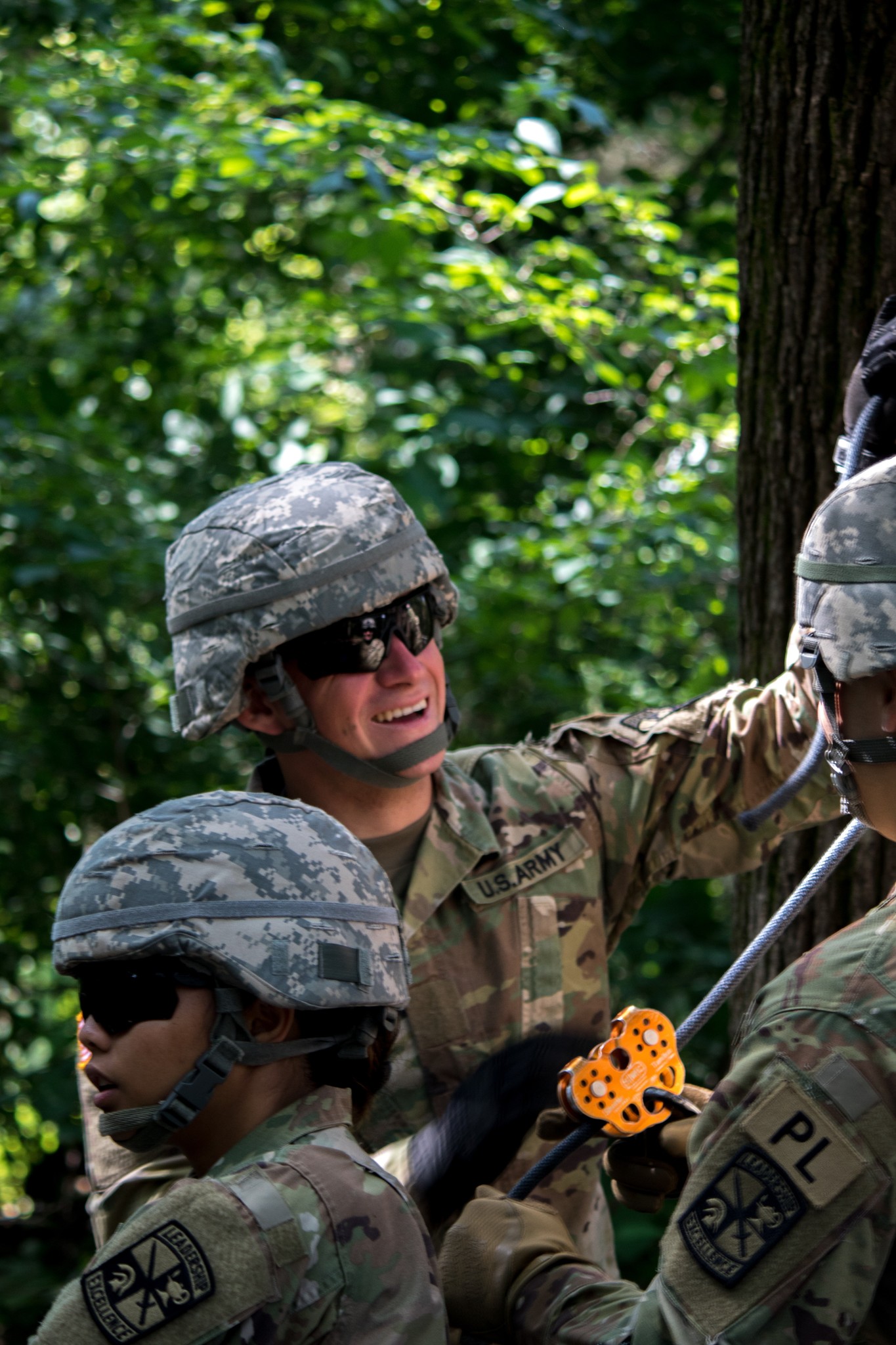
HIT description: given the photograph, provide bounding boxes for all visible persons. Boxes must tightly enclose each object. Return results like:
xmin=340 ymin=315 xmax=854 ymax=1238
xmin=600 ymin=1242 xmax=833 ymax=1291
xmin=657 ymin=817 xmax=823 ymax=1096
xmin=32 ymin=787 xmax=448 ymax=1345
xmin=437 ymin=459 xmax=896 ymax=1345
xmin=78 ymin=293 xmax=896 ymax=1281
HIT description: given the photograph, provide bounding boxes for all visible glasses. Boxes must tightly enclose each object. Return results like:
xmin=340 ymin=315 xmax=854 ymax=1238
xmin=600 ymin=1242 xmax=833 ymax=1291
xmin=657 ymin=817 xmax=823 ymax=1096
xmin=79 ymin=958 xmax=227 ymax=1039
xmin=280 ymin=582 xmax=453 ymax=678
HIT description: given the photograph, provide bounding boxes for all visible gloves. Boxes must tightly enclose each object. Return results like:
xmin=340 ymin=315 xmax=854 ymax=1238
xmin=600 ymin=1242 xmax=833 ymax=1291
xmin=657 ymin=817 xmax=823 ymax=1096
xmin=536 ymin=1084 xmax=714 ymax=1215
xmin=407 ymin=1023 xmax=609 ymax=1225
xmin=844 ymin=292 xmax=896 ymax=456
xmin=434 ymin=1182 xmax=575 ymax=1316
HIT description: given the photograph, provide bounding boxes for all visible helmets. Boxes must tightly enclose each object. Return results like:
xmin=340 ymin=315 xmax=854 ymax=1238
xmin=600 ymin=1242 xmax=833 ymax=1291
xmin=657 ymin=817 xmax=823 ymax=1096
xmin=52 ymin=792 xmax=414 ymax=1010
xmin=792 ymin=454 xmax=896 ymax=677
xmin=161 ymin=461 xmax=450 ymax=744
